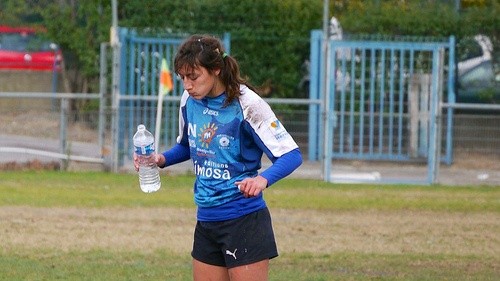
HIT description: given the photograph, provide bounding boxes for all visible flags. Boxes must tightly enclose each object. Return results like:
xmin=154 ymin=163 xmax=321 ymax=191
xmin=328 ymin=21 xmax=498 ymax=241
xmin=161 ymin=58 xmax=176 ymax=97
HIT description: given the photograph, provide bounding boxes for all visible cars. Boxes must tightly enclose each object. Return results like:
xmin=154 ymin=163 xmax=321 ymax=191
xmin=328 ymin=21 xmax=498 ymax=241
xmin=0 ymin=25 xmax=63 ymax=69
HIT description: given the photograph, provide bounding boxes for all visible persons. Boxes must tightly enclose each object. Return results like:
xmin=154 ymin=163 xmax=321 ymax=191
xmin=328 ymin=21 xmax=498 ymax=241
xmin=134 ymin=34 xmax=304 ymax=281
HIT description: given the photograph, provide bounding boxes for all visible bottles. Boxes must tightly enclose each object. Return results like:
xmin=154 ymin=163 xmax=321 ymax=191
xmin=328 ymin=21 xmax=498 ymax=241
xmin=133 ymin=124 xmax=161 ymax=194
